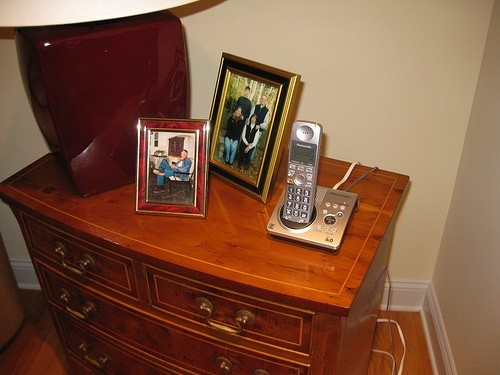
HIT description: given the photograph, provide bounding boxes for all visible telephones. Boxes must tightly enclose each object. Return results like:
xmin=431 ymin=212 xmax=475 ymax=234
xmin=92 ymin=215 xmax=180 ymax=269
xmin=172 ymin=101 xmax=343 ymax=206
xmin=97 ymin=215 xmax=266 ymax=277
xmin=266 ymin=120 xmax=358 ymax=250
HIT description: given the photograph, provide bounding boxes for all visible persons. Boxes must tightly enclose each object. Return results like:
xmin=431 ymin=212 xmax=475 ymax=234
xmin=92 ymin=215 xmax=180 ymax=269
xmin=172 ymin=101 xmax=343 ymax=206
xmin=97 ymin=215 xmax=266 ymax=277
xmin=232 ymin=86 xmax=251 ymax=119
xmin=245 ymin=95 xmax=270 ymax=140
xmin=152 ymin=150 xmax=192 ymax=193
xmin=224 ymin=105 xmax=245 ymax=165
xmin=238 ymin=113 xmax=259 ymax=170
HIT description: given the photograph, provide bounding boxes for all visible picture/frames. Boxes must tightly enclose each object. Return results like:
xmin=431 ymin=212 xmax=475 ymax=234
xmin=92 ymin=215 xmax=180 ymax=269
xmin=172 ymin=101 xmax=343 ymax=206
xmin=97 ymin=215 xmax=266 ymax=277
xmin=135 ymin=117 xmax=210 ymax=218
xmin=209 ymin=51 xmax=304 ymax=204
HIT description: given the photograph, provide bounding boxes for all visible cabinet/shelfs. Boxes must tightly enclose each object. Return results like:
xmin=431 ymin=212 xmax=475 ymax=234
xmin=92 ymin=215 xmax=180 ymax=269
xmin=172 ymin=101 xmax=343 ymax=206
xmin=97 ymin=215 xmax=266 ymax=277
xmin=0 ymin=148 xmax=410 ymax=375
